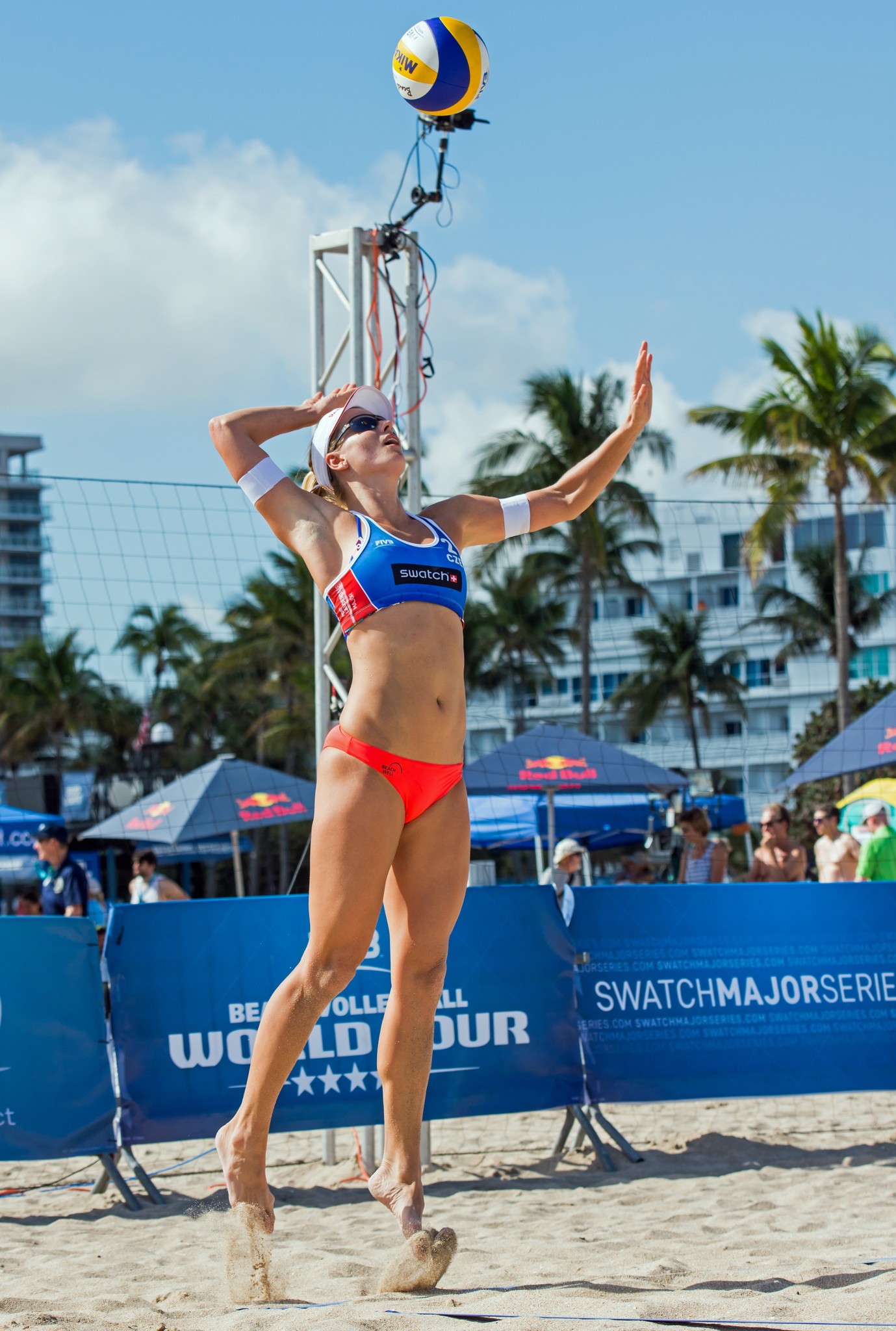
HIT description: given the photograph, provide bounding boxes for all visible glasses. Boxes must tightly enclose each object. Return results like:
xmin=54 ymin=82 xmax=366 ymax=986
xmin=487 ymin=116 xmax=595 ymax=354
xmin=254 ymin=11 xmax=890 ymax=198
xmin=817 ymin=819 xmax=823 ymax=822
xmin=38 ymin=836 xmax=48 ymax=842
xmin=574 ymin=853 xmax=580 ymax=856
xmin=331 ymin=412 xmax=384 ymax=450
xmin=759 ymin=818 xmax=782 ymax=827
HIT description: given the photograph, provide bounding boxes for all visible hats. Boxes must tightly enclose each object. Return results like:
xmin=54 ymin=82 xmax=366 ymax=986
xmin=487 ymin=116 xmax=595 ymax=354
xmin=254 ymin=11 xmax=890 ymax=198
xmin=621 ymin=853 xmax=656 ymax=872
xmin=552 ymin=838 xmax=585 ymax=864
xmin=861 ymin=804 xmax=886 ymax=823
xmin=34 ymin=822 xmax=68 ymax=843
xmin=310 ymin=386 xmax=393 ymax=495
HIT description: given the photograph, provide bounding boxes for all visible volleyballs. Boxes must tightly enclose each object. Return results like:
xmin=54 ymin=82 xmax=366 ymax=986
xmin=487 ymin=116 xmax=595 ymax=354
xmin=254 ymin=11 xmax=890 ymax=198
xmin=392 ymin=16 xmax=490 ymax=117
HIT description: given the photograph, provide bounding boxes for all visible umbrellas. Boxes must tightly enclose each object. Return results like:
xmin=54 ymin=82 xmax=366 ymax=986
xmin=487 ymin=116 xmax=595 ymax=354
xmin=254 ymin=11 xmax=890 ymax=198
xmin=79 ymin=754 xmax=315 ymax=897
xmin=779 ymin=689 xmax=896 ymax=786
xmin=462 ymin=723 xmax=690 ymax=866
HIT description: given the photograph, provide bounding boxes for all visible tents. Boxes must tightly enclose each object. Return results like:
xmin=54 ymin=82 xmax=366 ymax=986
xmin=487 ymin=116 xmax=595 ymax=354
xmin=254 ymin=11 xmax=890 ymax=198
xmin=467 ymin=794 xmax=756 ymax=886
xmin=0 ymin=805 xmax=66 ymax=856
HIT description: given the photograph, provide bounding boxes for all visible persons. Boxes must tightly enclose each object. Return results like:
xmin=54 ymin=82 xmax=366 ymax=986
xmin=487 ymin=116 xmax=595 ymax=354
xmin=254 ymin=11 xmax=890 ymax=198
xmin=538 ymin=804 xmax=896 ymax=885
xmin=217 ymin=342 xmax=654 ymax=1241
xmin=33 ymin=821 xmax=192 ymax=949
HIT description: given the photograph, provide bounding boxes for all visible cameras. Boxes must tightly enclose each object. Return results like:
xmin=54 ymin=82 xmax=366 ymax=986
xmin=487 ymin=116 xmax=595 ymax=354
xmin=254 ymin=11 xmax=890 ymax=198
xmin=433 ymin=109 xmax=476 ymax=129
